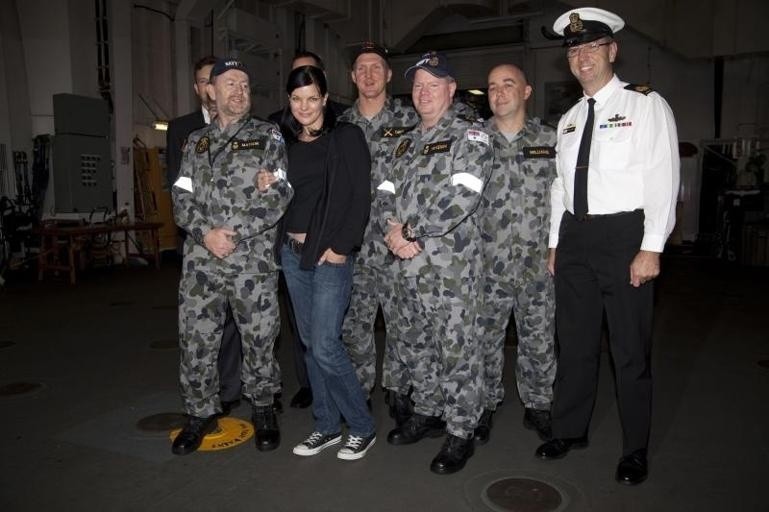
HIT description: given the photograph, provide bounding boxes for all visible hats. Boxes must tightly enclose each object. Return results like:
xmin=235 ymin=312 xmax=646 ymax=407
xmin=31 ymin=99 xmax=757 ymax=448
xmin=552 ymin=6 xmax=626 ymax=48
xmin=405 ymin=52 xmax=456 ymax=82
xmin=212 ymin=57 xmax=249 ymax=78
xmin=350 ymin=42 xmax=391 ymax=70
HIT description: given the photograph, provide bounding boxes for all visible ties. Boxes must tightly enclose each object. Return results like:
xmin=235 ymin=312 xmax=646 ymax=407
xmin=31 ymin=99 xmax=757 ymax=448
xmin=573 ymin=97 xmax=597 ymax=217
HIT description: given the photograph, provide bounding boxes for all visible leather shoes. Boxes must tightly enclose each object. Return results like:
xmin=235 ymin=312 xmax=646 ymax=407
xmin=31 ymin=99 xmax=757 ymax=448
xmin=250 ymin=399 xmax=282 ymax=452
xmin=386 ymin=395 xmax=491 ymax=476
xmin=615 ymin=438 xmax=649 ymax=487
xmin=523 ymin=406 xmax=590 ymax=461
xmin=171 ymin=411 xmax=219 ymax=457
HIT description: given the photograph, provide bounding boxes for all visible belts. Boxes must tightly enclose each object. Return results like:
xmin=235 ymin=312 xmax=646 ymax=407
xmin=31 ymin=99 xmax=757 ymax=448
xmin=283 ymin=235 xmax=304 ymax=252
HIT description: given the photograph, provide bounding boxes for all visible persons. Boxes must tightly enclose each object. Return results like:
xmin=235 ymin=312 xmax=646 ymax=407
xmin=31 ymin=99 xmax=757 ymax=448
xmin=472 ymin=63 xmax=557 ymax=448
xmin=534 ymin=5 xmax=680 ymax=485
xmin=164 ymin=55 xmax=285 ymax=417
xmin=265 ymin=49 xmax=353 ymax=409
xmin=172 ymin=56 xmax=296 ymax=456
xmin=255 ymin=64 xmax=379 ymax=461
xmin=376 ymin=58 xmax=496 ymax=477
xmin=336 ymin=41 xmax=421 ymax=428
xmin=421 ymin=47 xmax=481 ymax=123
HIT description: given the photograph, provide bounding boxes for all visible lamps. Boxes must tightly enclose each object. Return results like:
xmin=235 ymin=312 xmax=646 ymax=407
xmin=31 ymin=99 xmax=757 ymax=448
xmin=152 ymin=120 xmax=168 ymax=131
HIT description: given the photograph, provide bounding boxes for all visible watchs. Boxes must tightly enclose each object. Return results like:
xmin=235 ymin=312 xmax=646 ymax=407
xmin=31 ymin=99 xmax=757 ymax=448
xmin=401 ymin=223 xmax=416 ymax=242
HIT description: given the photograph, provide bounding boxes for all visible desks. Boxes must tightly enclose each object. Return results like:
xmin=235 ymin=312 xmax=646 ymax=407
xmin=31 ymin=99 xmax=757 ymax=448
xmin=37 ymin=222 xmax=163 ymax=284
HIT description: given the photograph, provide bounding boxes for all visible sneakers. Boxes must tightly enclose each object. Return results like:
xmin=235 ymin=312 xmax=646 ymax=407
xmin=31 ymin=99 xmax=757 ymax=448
xmin=292 ymin=428 xmax=343 ymax=457
xmin=289 ymin=373 xmax=322 ymax=410
xmin=336 ymin=428 xmax=378 ymax=461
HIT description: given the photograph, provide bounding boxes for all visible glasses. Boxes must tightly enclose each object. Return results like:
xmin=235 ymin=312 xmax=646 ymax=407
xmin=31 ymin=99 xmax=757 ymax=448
xmin=566 ymin=42 xmax=611 ymax=58
